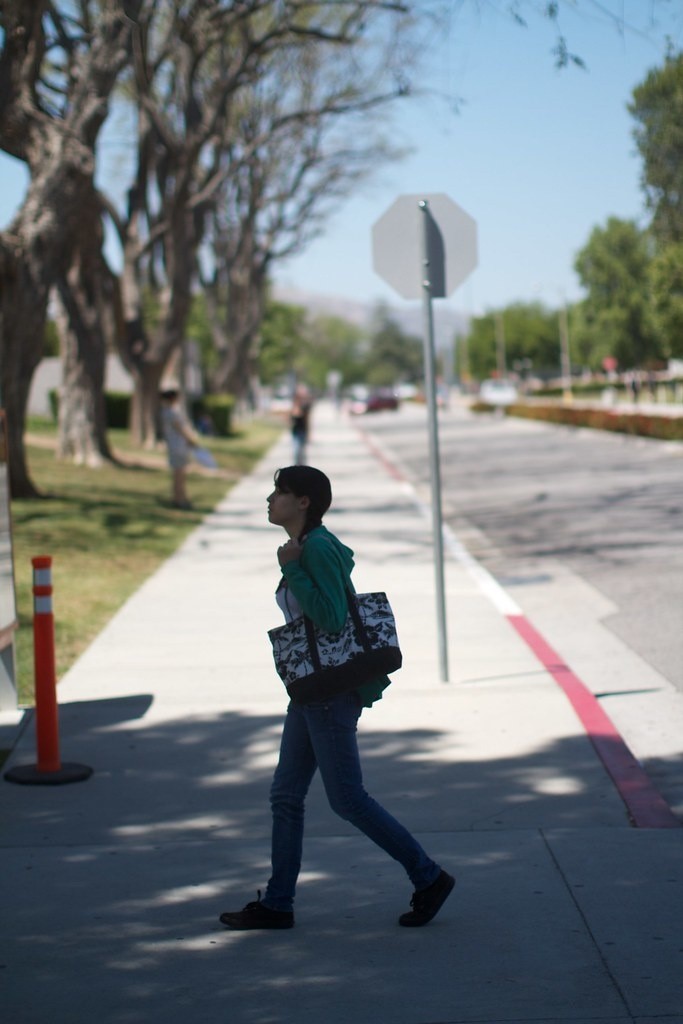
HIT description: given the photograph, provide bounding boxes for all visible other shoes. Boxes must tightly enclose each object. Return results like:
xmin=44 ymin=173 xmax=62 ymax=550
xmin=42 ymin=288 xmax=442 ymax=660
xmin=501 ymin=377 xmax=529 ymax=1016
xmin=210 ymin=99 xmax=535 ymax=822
xmin=174 ymin=500 xmax=192 ymax=510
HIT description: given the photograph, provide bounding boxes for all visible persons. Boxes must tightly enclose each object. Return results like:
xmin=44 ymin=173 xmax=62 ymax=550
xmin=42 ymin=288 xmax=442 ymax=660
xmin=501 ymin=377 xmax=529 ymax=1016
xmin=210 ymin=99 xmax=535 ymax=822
xmin=289 ymin=384 xmax=311 ymax=467
xmin=159 ymin=389 xmax=204 ymax=513
xmin=218 ymin=464 xmax=456 ymax=928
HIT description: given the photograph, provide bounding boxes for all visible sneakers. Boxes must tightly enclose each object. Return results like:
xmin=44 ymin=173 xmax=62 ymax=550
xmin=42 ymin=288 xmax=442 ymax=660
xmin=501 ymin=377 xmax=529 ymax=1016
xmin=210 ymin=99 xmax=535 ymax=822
xmin=219 ymin=889 xmax=295 ymax=930
xmin=399 ymin=868 xmax=455 ymax=927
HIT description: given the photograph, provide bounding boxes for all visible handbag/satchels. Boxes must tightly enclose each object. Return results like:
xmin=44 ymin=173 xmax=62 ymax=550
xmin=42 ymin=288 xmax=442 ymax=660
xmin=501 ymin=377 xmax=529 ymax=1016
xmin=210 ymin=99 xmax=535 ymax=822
xmin=267 ymin=533 xmax=402 ymax=706
